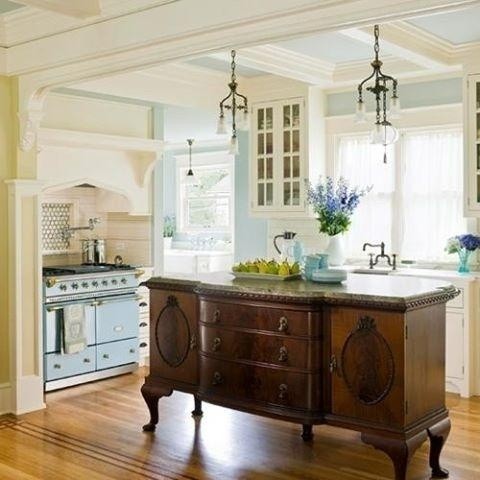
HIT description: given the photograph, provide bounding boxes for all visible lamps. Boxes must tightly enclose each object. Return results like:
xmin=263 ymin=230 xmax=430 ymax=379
xmin=216 ymin=49 xmax=252 ymax=156
xmin=354 ymin=25 xmax=403 ymax=163
xmin=180 ymin=138 xmax=199 ymax=184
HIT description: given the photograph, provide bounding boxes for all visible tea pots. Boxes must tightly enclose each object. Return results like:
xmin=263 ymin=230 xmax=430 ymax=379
xmin=273 ymin=232 xmax=298 ymax=262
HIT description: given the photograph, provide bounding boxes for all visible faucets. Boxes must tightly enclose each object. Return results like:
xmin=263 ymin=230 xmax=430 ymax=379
xmin=65 ymin=216 xmax=101 ymax=238
xmin=363 ymin=242 xmax=382 ymax=251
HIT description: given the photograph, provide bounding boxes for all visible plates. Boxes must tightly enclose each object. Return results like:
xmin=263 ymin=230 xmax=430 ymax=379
xmin=312 ymin=269 xmax=348 ymax=283
xmin=284 ymin=190 xmax=301 ymax=205
xmin=229 ymin=270 xmax=303 ymax=280
xmin=258 ymin=119 xmax=272 ymax=128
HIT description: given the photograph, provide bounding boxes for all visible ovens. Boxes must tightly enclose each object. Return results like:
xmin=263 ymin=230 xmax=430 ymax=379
xmin=40 ymin=293 xmax=140 ymax=381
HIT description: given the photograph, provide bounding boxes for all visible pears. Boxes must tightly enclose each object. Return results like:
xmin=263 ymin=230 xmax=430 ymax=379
xmin=233 ymin=258 xmax=300 ymax=276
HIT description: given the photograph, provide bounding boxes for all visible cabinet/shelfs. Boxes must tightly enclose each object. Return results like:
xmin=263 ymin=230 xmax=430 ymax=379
xmin=250 ymin=97 xmax=326 ymax=220
xmin=137 ymin=267 xmax=153 ymax=365
xmin=445 ymin=286 xmax=480 ymax=379
xmin=463 ymin=73 xmax=480 ymax=217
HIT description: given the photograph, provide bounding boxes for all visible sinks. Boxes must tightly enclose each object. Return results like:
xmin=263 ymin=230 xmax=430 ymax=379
xmin=348 ymin=269 xmax=389 ymax=275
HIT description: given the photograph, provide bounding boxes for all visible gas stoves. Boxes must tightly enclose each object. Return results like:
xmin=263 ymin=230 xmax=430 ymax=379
xmin=42 ymin=263 xmax=138 ymax=297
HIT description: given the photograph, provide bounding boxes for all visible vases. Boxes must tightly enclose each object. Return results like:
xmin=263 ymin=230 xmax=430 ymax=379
xmin=164 ymin=237 xmax=172 ymax=249
xmin=457 ymin=250 xmax=471 ymax=273
xmin=324 ymin=232 xmax=346 ymax=266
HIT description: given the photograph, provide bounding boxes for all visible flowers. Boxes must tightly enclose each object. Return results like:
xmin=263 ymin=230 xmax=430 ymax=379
xmin=303 ymin=175 xmax=375 ymax=237
xmin=444 ymin=234 xmax=480 ymax=254
xmin=164 ymin=215 xmax=176 ymax=238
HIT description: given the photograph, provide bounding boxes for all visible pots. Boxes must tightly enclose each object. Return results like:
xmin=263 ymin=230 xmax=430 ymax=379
xmin=79 ymin=240 xmax=106 ymax=264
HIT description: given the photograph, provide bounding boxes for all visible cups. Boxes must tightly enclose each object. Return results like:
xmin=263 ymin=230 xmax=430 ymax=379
xmin=301 ymin=254 xmax=328 ymax=279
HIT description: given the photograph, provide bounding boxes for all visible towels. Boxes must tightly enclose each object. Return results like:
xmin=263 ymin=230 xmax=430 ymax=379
xmin=59 ymin=304 xmax=88 ymax=356
